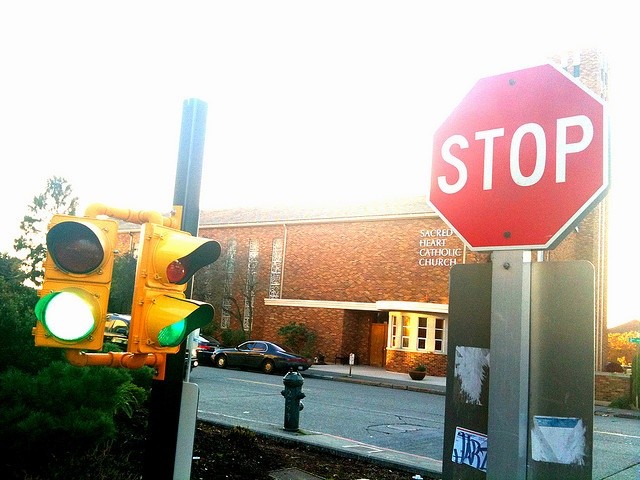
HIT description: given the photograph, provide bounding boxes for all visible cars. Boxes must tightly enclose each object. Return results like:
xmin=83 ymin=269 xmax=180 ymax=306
xmin=210 ymin=341 xmax=308 ymax=373
xmin=196 ymin=333 xmax=222 ymax=365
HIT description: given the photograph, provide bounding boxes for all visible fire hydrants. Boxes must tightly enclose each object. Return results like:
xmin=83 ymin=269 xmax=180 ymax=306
xmin=281 ymin=368 xmax=306 ymax=432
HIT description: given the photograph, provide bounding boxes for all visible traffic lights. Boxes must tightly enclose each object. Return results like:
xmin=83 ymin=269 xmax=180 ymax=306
xmin=32 ymin=215 xmax=118 ymax=350
xmin=128 ymin=223 xmax=221 ymax=354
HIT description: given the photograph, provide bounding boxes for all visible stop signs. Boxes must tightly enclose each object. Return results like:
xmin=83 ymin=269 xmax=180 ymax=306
xmin=429 ymin=64 xmax=610 ymax=252
